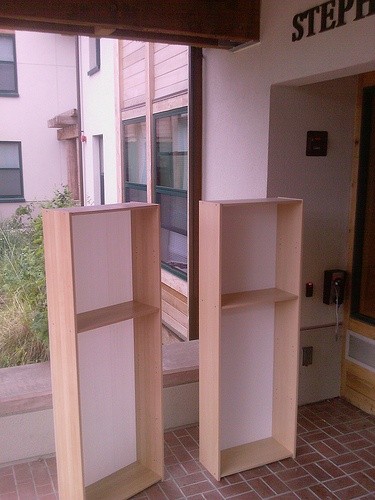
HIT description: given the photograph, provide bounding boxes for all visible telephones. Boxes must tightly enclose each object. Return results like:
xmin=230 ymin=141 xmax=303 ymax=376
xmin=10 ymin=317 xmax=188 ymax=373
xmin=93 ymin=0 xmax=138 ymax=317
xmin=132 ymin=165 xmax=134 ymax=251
xmin=324 ymin=269 xmax=350 ymax=304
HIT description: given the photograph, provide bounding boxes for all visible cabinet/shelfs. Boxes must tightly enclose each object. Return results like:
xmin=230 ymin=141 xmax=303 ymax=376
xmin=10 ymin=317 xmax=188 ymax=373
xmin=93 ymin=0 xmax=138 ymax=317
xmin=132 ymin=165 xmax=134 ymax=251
xmin=42 ymin=202 xmax=167 ymax=500
xmin=339 ymin=72 xmax=374 ymax=418
xmin=197 ymin=198 xmax=304 ymax=481
